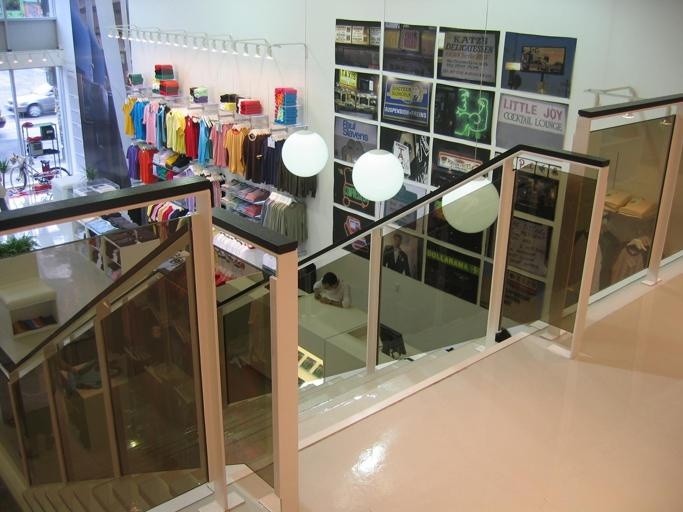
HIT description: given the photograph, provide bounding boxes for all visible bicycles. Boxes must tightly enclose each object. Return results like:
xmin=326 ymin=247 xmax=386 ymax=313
xmin=9 ymin=153 xmax=70 ymax=192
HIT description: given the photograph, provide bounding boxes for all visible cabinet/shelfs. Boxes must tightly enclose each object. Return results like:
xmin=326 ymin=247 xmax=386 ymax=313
xmin=72 ymin=212 xmax=161 ymax=282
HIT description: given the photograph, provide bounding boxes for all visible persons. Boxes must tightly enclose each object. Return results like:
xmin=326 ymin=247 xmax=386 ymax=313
xmin=383 ymin=233 xmax=411 ymax=277
xmin=313 ymin=272 xmax=350 ymax=310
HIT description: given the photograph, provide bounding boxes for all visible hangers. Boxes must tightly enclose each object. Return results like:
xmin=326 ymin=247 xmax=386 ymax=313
xmin=148 ymin=199 xmax=189 ymax=219
xmin=122 ymin=87 xmax=296 ymax=157
xmin=262 ymin=186 xmax=306 ymax=213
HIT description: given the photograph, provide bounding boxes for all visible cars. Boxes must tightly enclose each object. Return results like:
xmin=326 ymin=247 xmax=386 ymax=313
xmin=6 ymin=82 xmax=55 ymax=117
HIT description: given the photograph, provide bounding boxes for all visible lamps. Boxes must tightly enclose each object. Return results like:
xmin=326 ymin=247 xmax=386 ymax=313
xmin=351 ymin=1 xmax=404 ymax=202
xmin=441 ymin=2 xmax=500 ymax=234
xmin=281 ymin=0 xmax=329 ymax=178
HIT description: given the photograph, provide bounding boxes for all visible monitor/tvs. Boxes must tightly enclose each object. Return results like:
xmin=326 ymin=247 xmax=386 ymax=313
xmin=380 ymin=323 xmax=407 ymax=361
xmin=262 ymin=264 xmax=277 ymax=290
